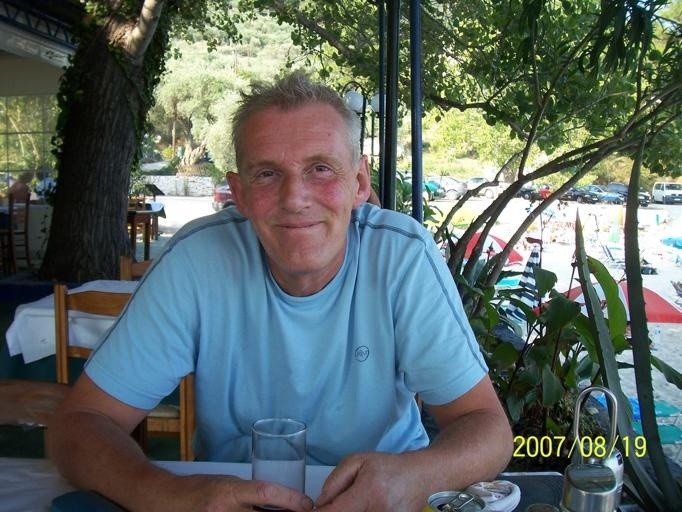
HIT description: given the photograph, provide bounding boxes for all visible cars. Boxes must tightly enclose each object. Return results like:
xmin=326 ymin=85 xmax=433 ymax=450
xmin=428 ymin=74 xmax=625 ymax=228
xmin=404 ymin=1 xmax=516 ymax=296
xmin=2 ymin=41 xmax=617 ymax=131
xmin=420 ymin=176 xmax=682 ymax=207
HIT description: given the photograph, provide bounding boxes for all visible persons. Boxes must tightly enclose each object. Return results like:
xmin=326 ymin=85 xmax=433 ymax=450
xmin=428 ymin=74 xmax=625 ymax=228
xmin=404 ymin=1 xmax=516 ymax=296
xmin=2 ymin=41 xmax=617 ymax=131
xmin=44 ymin=63 xmax=524 ymax=512
xmin=32 ymin=165 xmax=58 ymax=200
xmin=6 ymin=167 xmax=33 ymax=204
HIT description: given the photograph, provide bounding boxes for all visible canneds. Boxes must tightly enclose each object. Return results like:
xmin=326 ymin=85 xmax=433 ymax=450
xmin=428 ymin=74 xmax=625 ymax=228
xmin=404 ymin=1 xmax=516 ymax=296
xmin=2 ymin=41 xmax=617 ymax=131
xmin=422 ymin=490 xmax=487 ymax=512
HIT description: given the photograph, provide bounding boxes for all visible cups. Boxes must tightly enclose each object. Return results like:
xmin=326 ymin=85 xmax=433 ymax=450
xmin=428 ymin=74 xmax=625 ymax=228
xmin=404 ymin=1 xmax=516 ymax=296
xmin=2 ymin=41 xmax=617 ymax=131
xmin=252 ymin=417 xmax=307 ymax=511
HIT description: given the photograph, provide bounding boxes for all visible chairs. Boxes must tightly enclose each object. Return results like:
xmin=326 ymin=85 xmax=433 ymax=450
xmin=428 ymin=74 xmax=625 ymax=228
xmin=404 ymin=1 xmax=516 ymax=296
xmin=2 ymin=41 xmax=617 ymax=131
xmin=587 ymin=392 xmax=682 ymax=462
xmin=54 ymin=284 xmax=196 ymax=461
xmin=0 ymin=193 xmax=32 ymax=274
xmin=0 ymin=381 xmax=147 ymax=460
xmin=120 ymin=254 xmax=155 ymax=280
